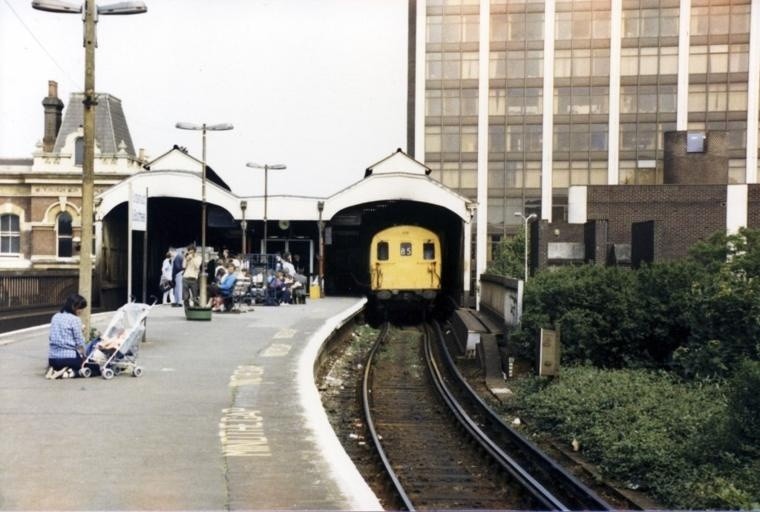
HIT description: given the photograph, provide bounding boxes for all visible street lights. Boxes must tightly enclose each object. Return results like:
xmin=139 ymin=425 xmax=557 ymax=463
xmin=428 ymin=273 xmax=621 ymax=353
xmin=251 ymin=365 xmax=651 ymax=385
xmin=511 ymin=209 xmax=539 ymax=282
xmin=172 ymin=120 xmax=236 ymax=308
xmin=238 ymin=199 xmax=250 ymax=255
xmin=244 ymin=161 xmax=287 ymax=306
xmin=27 ymin=0 xmax=152 ymax=346
xmin=316 ymin=199 xmax=328 ymax=298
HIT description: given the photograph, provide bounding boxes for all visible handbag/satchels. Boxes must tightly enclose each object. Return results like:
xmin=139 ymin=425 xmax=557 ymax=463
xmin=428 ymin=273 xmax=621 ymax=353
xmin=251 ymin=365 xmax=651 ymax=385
xmin=159 ymin=280 xmax=175 ymax=292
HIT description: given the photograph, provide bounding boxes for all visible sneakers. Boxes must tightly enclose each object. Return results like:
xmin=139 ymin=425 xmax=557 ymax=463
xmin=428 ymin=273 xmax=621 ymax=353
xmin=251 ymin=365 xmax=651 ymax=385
xmin=45 ymin=366 xmax=68 ymax=379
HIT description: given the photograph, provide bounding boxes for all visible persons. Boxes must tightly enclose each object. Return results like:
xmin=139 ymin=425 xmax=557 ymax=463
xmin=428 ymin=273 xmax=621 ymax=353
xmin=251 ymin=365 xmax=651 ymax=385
xmin=46 ymin=295 xmax=127 ymax=379
xmin=159 ymin=245 xmax=301 ymax=312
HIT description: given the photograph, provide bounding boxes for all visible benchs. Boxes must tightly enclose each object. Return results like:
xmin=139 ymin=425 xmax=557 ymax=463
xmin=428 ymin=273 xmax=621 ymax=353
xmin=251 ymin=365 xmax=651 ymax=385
xmin=217 ymin=275 xmax=310 ymax=304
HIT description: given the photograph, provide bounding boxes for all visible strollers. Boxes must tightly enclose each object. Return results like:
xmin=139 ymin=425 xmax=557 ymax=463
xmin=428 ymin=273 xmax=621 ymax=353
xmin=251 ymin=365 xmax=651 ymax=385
xmin=74 ymin=292 xmax=163 ymax=381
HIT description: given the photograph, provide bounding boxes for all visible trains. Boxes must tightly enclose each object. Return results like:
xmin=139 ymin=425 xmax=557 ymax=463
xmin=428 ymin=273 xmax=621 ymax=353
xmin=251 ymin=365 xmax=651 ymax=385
xmin=359 ymin=219 xmax=443 ymax=325
